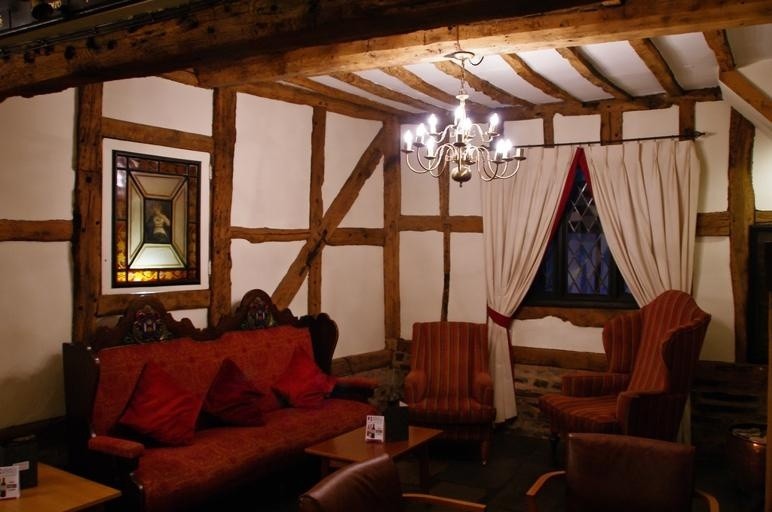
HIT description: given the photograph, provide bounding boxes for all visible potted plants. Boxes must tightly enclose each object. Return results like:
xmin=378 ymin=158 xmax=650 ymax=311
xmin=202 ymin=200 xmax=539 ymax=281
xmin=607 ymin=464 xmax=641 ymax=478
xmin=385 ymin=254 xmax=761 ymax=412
xmin=368 ymin=368 xmax=408 ymax=442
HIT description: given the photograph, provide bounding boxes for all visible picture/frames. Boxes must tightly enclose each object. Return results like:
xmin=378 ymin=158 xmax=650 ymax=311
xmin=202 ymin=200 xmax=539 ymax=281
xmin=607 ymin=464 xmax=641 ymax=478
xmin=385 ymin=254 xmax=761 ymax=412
xmin=113 ymin=150 xmax=200 ymax=288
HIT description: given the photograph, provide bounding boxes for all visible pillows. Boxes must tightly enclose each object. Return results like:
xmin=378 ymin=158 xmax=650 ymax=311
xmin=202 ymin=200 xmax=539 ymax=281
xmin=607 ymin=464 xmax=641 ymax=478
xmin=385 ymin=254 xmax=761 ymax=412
xmin=119 ymin=362 xmax=208 ymax=446
xmin=265 ymin=345 xmax=337 ymax=414
xmin=203 ymin=357 xmax=281 ymax=426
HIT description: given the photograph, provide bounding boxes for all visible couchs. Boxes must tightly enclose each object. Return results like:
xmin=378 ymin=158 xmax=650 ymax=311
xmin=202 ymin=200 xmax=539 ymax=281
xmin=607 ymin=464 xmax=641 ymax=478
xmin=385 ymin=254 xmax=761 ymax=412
xmin=63 ymin=289 xmax=383 ymax=512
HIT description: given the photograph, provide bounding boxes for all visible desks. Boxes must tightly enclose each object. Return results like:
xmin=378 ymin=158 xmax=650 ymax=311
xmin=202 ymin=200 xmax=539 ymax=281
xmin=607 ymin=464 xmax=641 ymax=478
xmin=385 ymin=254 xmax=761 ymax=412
xmin=0 ymin=462 xmax=121 ymax=512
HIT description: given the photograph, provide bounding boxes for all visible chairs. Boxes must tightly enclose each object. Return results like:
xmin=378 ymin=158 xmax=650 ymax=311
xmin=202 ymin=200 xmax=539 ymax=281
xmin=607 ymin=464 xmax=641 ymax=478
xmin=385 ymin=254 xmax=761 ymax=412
xmin=298 ymin=453 xmax=488 ymax=512
xmin=538 ymin=291 xmax=710 ymax=448
xmin=403 ymin=322 xmax=496 ymax=467
xmin=525 ymin=433 xmax=720 ymax=512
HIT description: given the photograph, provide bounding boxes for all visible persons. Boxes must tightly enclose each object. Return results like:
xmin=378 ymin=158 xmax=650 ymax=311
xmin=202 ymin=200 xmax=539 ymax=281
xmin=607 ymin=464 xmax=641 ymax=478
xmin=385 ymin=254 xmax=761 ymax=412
xmin=147 ymin=202 xmax=171 ymax=244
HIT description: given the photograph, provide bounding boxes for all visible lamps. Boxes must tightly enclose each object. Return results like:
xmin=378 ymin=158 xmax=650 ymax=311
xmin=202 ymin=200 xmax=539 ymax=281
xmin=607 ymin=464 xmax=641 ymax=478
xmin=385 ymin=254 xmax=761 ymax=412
xmin=402 ymin=51 xmax=526 ymax=187
